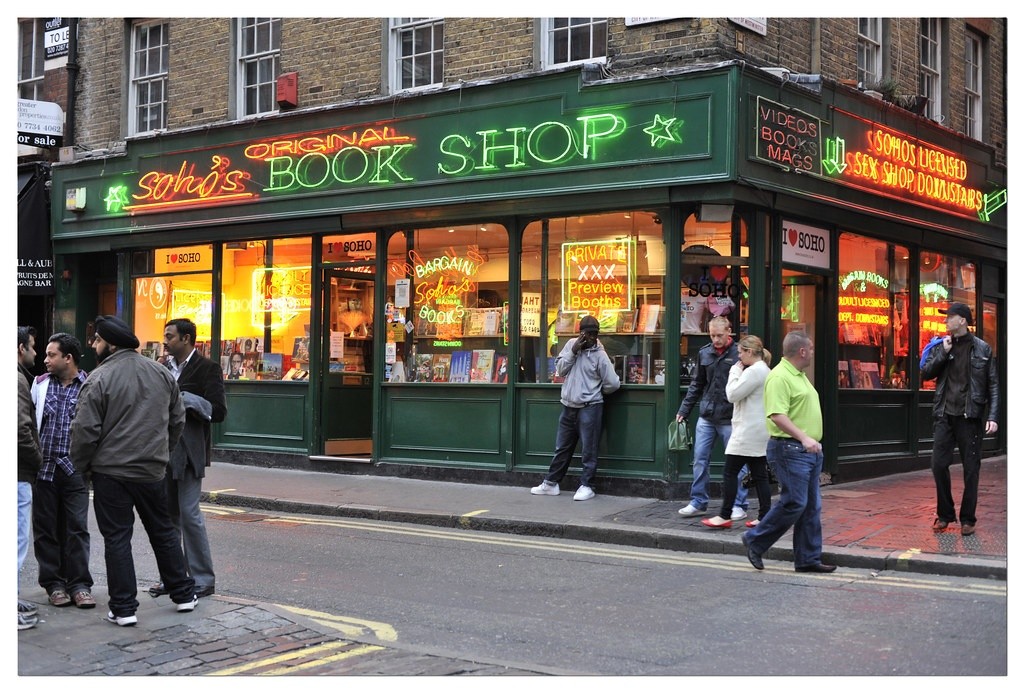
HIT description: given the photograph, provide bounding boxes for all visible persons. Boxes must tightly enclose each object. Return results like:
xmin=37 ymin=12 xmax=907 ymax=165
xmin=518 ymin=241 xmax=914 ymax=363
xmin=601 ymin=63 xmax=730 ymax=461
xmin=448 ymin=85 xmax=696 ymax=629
xmin=150 ymin=319 xmax=227 ymax=599
xmin=531 ymin=316 xmax=621 ymax=501
xmin=28 ymin=333 xmax=96 ymax=609
xmin=226 ymin=352 xmax=243 ymax=380
xmin=69 ymin=318 xmax=198 ymax=626
xmin=739 ymin=332 xmax=837 ymax=572
xmin=18 ymin=326 xmax=42 ymax=632
xmin=919 ymin=303 xmax=999 ymax=535
xmin=243 ymin=339 xmax=252 ymax=356
xmin=675 ymin=315 xmax=749 ymax=521
xmin=839 ymin=371 xmax=851 ymax=388
xmin=699 ymin=334 xmax=772 ymax=528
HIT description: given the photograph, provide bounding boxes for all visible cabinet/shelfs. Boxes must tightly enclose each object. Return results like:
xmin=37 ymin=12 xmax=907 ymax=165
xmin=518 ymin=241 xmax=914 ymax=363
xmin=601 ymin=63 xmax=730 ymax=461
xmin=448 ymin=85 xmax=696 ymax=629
xmin=546 ymin=284 xmax=663 ymax=385
xmin=386 ymin=306 xmax=510 ymax=383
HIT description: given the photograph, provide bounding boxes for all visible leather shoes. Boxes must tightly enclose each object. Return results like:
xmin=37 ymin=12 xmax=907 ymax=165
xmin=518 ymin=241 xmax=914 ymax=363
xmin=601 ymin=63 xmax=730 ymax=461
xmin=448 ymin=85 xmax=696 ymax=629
xmin=795 ymin=563 xmax=837 ymax=572
xmin=961 ymin=524 xmax=976 ymax=536
xmin=933 ymin=522 xmax=949 ymax=529
xmin=741 ymin=535 xmax=765 ymax=569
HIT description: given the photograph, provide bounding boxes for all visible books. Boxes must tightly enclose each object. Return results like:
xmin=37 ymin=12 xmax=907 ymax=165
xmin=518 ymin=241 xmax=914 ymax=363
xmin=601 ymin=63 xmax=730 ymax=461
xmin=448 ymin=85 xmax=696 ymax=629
xmin=413 ymin=305 xmax=515 ymax=384
xmin=141 ymin=335 xmax=310 ymax=380
xmin=838 ymin=358 xmax=881 ymax=390
xmin=552 ymin=304 xmax=668 ymax=383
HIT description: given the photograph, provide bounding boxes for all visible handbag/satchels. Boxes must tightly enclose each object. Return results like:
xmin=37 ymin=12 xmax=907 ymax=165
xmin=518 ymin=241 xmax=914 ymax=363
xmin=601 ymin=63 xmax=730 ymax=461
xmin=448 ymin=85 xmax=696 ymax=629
xmin=919 ymin=336 xmax=946 ymax=369
xmin=668 ymin=418 xmax=693 ymax=453
xmin=741 ymin=462 xmax=775 ymax=489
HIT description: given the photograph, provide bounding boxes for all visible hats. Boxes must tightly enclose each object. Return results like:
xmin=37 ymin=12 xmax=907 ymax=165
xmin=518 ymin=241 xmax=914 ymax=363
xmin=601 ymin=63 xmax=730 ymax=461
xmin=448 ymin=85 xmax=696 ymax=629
xmin=95 ymin=314 xmax=140 ymax=348
xmin=938 ymin=303 xmax=972 ymax=322
xmin=579 ymin=316 xmax=599 ymax=333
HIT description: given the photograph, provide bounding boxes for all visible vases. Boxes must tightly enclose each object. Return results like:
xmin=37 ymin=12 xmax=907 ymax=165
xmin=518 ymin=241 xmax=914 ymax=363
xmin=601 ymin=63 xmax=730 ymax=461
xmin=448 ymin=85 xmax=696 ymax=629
xmin=838 ymin=79 xmax=857 ymax=87
xmin=903 ymin=96 xmax=930 ymax=113
xmin=864 ymin=90 xmax=884 ymax=101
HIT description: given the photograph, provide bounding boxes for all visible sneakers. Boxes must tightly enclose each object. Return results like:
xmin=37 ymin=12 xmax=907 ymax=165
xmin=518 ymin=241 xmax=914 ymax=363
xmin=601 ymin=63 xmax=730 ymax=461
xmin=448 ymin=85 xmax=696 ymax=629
xmin=573 ymin=485 xmax=596 ymax=500
xmin=107 ymin=611 xmax=138 ymax=626
xmin=176 ymin=594 xmax=199 ymax=612
xmin=678 ymin=505 xmax=708 ymax=517
xmin=530 ymin=479 xmax=560 ymax=496
xmin=730 ymin=508 xmax=748 ymax=521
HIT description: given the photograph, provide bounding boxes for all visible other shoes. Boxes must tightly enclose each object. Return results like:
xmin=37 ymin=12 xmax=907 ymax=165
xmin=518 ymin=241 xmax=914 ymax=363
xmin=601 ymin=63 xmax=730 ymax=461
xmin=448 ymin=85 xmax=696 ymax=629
xmin=48 ymin=590 xmax=71 ymax=607
xmin=18 ymin=600 xmax=38 ymax=617
xmin=701 ymin=519 xmax=732 ymax=528
xmin=194 ymin=586 xmax=215 ymax=597
xmin=18 ymin=617 xmax=39 ymax=630
xmin=745 ymin=521 xmax=756 ymax=527
xmin=74 ymin=592 xmax=96 ymax=608
xmin=149 ymin=582 xmax=167 ymax=594
